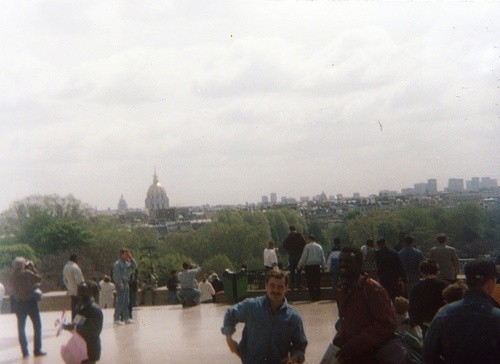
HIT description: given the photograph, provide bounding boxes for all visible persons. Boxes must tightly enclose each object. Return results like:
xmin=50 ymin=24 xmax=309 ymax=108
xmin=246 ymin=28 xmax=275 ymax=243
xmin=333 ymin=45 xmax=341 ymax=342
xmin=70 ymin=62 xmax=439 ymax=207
xmin=328 ymin=233 xmax=500 ymax=364
xmin=141 ymin=267 xmax=159 ymax=305
xmin=263 ymin=241 xmax=279 ymax=270
xmin=167 ymin=262 xmax=249 ymax=306
xmin=283 ymin=226 xmax=306 ymax=289
xmin=298 ymin=235 xmax=327 ymax=300
xmin=221 ymin=269 xmax=307 ymax=364
xmin=111 ymin=248 xmax=139 ymax=325
xmin=89 ymin=276 xmax=116 ymax=308
xmin=9 ymin=256 xmax=47 ymax=359
xmin=62 ymin=255 xmax=85 ymax=320
xmin=59 ymin=281 xmax=103 ymax=364
xmin=0 ymin=283 xmax=5 ymax=313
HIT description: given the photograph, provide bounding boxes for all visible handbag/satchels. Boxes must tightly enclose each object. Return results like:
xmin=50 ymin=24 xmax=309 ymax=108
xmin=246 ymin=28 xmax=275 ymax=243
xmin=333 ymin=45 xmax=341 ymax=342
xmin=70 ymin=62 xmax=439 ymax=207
xmin=60 ymin=331 xmax=89 ymax=364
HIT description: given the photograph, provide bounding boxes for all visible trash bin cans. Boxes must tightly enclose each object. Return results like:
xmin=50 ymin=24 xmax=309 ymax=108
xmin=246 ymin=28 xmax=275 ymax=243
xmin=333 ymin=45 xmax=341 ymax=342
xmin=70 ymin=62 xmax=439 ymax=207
xmin=222 ymin=268 xmax=247 ymax=303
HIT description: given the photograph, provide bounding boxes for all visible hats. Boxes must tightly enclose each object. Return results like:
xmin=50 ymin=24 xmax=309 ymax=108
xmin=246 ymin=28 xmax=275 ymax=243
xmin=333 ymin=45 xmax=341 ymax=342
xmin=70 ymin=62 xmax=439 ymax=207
xmin=464 ymin=257 xmax=497 ymax=284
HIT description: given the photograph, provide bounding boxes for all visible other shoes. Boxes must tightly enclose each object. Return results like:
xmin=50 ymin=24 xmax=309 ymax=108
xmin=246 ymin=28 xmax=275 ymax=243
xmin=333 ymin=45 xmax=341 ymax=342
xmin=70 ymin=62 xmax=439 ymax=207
xmin=123 ymin=319 xmax=133 ymax=324
xmin=114 ymin=319 xmax=124 ymax=326
